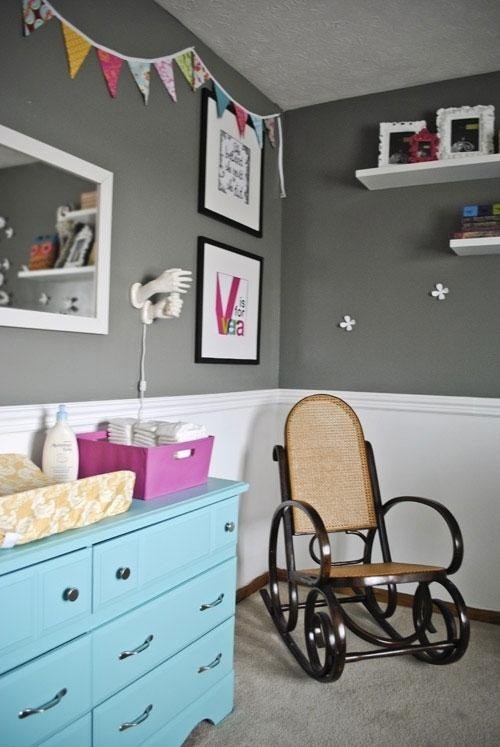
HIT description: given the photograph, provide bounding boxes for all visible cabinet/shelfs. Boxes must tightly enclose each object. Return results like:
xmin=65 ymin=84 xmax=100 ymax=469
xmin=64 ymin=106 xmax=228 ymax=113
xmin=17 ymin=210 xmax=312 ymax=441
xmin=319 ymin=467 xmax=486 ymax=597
xmin=16 ymin=205 xmax=96 ymax=278
xmin=0 ymin=491 xmax=243 ymax=747
xmin=357 ymin=144 xmax=500 ymax=258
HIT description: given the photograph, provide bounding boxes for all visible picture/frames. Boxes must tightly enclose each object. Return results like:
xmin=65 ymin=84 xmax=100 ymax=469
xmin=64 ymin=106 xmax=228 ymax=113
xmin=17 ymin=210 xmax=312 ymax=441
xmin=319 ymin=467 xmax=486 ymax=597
xmin=198 ymin=235 xmax=262 ymax=366
xmin=198 ymin=86 xmax=266 ymax=239
xmin=436 ymin=104 xmax=496 ymax=159
xmin=377 ymin=120 xmax=426 ymax=168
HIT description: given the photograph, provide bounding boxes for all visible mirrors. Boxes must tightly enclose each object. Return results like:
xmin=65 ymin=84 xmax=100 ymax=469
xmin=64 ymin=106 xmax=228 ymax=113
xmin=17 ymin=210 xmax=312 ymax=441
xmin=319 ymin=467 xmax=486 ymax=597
xmin=0 ymin=124 xmax=113 ymax=341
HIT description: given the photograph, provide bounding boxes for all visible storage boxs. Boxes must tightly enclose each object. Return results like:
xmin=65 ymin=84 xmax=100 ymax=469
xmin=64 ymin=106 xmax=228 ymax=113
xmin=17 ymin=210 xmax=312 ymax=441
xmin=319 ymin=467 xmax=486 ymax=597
xmin=77 ymin=430 xmax=215 ymax=503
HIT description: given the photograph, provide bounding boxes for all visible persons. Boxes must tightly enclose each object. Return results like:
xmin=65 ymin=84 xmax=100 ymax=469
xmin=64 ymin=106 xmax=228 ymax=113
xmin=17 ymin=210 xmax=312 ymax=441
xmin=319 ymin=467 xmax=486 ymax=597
xmin=129 ymin=267 xmax=194 ymax=325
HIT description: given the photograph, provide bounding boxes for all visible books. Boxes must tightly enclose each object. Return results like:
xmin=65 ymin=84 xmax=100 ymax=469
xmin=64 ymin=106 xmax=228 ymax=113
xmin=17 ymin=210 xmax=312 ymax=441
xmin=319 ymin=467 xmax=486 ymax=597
xmin=454 ymin=203 xmax=500 ymax=238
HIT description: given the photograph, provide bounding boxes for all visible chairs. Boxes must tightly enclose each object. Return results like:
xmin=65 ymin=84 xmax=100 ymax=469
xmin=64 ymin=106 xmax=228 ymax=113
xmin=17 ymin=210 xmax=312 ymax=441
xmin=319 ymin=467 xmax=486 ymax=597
xmin=261 ymin=393 xmax=474 ymax=681
